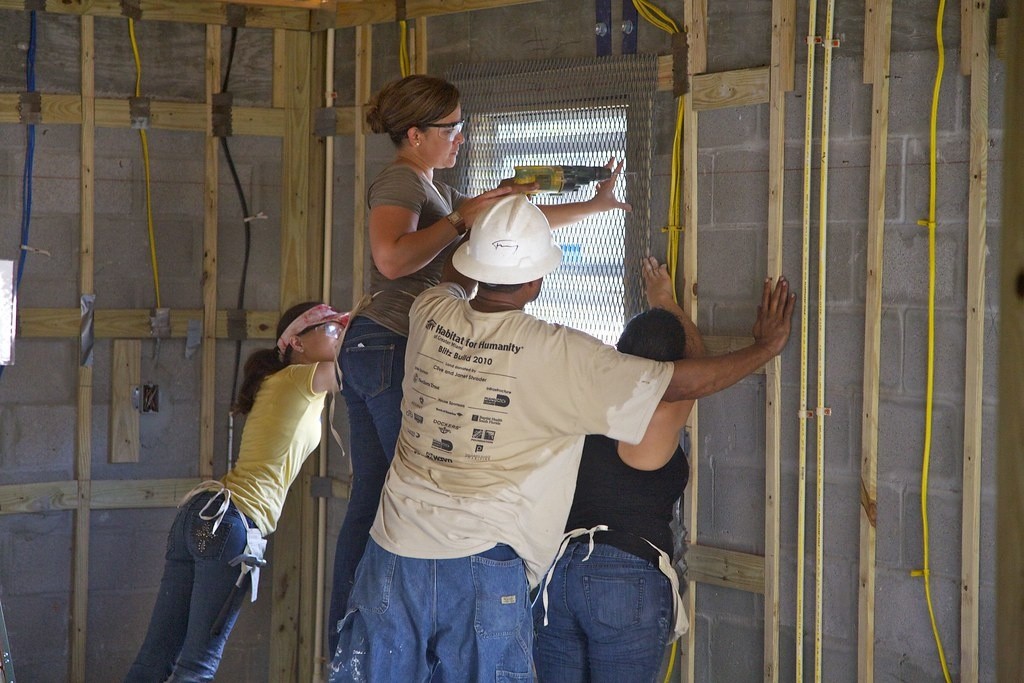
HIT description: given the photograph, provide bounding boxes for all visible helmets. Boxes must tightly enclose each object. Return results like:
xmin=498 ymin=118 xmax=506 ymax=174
xmin=452 ymin=193 xmax=563 ymax=284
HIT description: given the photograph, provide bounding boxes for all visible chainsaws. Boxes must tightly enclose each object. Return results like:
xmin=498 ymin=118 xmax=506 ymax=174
xmin=515 ymin=165 xmax=639 ymax=194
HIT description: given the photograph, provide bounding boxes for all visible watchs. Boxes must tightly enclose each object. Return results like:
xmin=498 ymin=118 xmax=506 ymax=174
xmin=447 ymin=211 xmax=466 ymax=236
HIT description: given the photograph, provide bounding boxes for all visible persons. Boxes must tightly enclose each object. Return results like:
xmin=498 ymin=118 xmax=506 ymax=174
xmin=328 ymin=157 xmax=797 ymax=683
xmin=327 ymin=76 xmax=631 ymax=657
xmin=530 ymin=256 xmax=704 ymax=683
xmin=124 ymin=302 xmax=350 ymax=683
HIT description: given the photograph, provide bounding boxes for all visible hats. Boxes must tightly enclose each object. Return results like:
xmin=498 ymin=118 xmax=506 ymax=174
xmin=277 ymin=304 xmax=350 ymax=364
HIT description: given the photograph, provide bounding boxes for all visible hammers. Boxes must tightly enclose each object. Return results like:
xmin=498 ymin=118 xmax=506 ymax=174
xmin=209 ymin=554 xmax=266 ymax=642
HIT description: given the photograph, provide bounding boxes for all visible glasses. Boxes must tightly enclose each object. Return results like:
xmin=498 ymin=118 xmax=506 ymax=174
xmin=315 ymin=323 xmax=344 ymax=340
xmin=403 ymin=119 xmax=465 ymax=142
